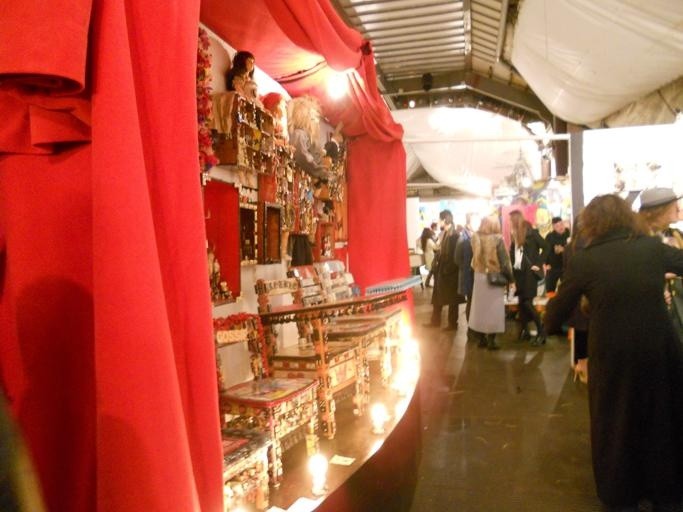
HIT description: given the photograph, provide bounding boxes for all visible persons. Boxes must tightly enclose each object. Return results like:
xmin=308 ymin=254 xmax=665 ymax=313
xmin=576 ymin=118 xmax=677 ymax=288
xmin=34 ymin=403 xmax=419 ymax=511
xmin=257 ymin=91 xmax=290 ymax=144
xmin=223 ymin=50 xmax=264 ymax=109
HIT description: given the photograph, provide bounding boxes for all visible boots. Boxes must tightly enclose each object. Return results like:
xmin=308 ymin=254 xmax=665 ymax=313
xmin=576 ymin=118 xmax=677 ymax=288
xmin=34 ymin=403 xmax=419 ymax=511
xmin=477 ymin=333 xmax=500 ymax=351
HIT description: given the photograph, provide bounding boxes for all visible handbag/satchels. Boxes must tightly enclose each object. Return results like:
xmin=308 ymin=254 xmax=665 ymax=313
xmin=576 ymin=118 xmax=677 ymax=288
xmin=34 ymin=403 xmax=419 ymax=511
xmin=533 ymin=267 xmax=544 ymax=280
xmin=489 ymin=271 xmax=507 ymax=286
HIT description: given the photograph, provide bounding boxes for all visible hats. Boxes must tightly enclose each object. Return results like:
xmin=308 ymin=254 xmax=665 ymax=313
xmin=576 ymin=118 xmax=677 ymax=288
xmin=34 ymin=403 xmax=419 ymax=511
xmin=638 ymin=187 xmax=683 ymax=210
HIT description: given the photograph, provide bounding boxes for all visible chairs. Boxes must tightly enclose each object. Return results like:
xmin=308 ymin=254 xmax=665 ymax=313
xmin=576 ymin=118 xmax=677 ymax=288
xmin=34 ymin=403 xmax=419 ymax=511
xmin=213 ymin=312 xmax=319 ymax=492
xmin=253 ymin=260 xmax=422 ymax=438
xmin=213 ymin=429 xmax=274 ymax=512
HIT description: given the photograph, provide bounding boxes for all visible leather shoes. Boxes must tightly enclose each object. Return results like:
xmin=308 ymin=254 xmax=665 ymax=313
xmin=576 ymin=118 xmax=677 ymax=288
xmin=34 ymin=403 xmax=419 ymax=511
xmin=422 ymin=322 xmax=441 ymax=328
xmin=441 ymin=326 xmax=457 ymax=331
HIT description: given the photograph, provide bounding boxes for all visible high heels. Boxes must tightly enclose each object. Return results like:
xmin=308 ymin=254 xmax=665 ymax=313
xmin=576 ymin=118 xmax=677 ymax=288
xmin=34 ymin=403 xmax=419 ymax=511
xmin=530 ymin=335 xmax=546 ymax=346
xmin=518 ymin=328 xmax=531 ymax=341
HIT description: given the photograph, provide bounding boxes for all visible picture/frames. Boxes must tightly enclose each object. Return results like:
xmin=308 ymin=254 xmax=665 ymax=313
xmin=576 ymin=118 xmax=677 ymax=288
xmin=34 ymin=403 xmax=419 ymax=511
xmin=240 ymin=201 xmax=259 ymax=267
xmin=263 ymin=201 xmax=283 ymax=265
xmin=201 ymin=172 xmax=244 ymax=309
